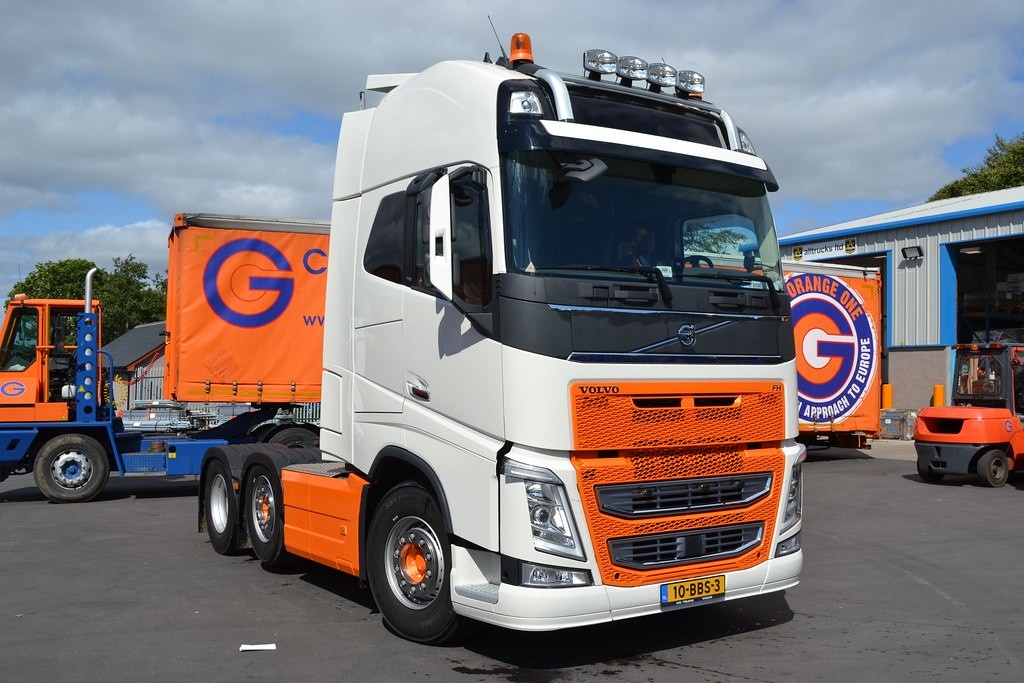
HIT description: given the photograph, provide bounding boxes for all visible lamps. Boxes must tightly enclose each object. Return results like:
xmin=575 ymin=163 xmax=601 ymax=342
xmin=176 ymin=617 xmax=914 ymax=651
xmin=901 ymin=244 xmax=923 ymax=260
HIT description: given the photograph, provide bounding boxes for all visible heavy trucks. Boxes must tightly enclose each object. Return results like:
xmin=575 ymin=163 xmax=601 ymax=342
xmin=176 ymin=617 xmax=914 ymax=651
xmin=0 ymin=214 xmax=884 ymax=503
xmin=198 ymin=32 xmax=807 ymax=645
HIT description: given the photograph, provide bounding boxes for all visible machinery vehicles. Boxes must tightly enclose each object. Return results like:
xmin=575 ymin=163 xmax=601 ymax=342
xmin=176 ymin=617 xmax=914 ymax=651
xmin=914 ymin=342 xmax=1023 ymax=488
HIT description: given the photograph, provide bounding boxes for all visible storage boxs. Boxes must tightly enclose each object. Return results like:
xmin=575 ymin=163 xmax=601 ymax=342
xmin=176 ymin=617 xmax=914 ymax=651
xmin=874 ymin=408 xmax=921 ymax=441
